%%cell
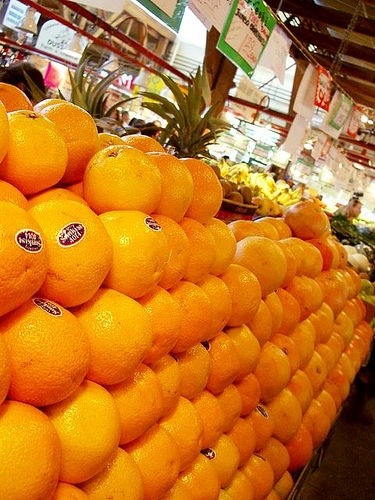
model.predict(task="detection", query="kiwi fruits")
[208,165,252,204]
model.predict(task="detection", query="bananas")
[208,159,327,208]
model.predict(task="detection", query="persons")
[103,109,160,141]
[0,63,47,107]
[298,182,309,200]
[334,198,361,223]
